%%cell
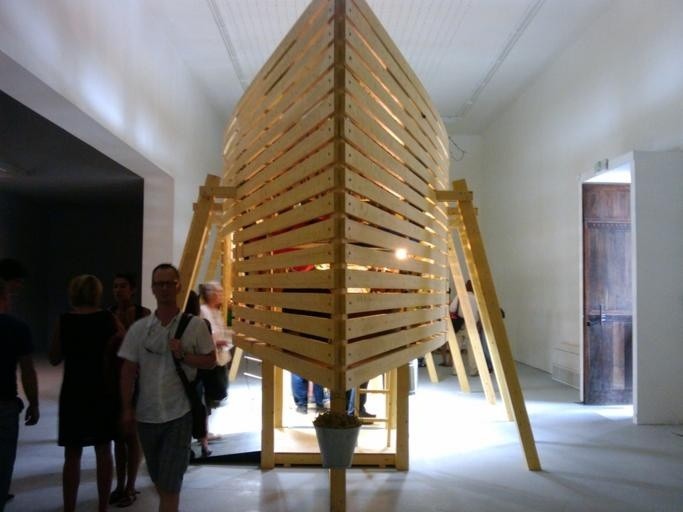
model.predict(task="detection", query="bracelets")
[178,349,185,360]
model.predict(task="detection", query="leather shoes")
[351,412,376,425]
[296,406,327,413]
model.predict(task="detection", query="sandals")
[110,488,137,507]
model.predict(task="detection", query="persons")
[0,282,39,512]
[185,279,236,462]
[289,370,330,415]
[346,378,377,425]
[474,319,492,373]
[450,279,482,377]
[114,262,218,512]
[96,272,150,510]
[43,274,126,512]
[436,286,456,367]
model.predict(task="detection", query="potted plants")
[313,412,363,469]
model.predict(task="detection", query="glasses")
[155,280,177,288]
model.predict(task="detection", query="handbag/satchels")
[451,314,464,333]
[185,378,208,414]
